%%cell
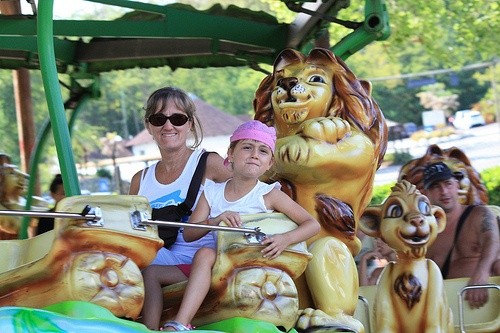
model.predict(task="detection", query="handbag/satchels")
[151,151,217,247]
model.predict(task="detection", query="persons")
[137,121,321,331]
[423,162,500,309]
[31,174,66,237]
[353,230,376,264]
[359,238,398,286]
[127,87,233,279]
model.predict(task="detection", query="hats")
[424,162,451,190]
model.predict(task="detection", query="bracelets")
[207,218,211,225]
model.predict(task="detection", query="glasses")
[146,113,191,126]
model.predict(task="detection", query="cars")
[447,110,485,129]
[384,119,437,140]
